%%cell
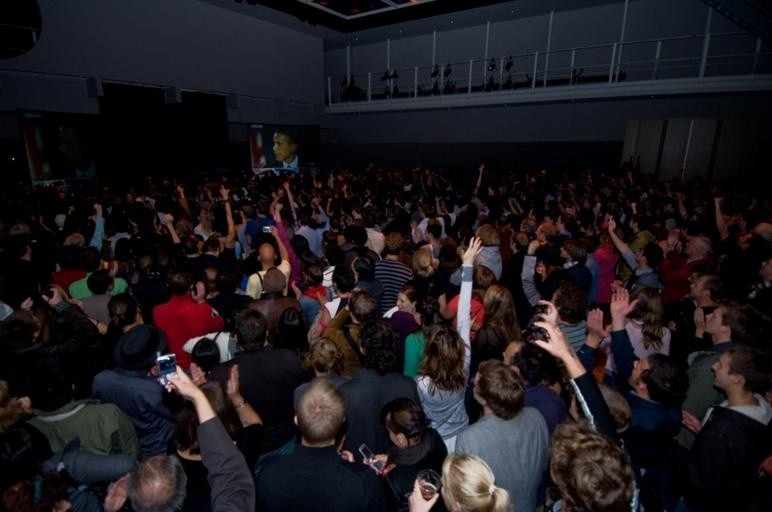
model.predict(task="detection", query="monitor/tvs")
[247,122,321,175]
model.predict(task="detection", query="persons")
[1,167,770,511]
[273,127,309,170]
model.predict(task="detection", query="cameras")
[518,305,546,344]
[263,226,272,233]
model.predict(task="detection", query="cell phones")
[610,215,614,224]
[156,353,178,386]
[358,443,386,474]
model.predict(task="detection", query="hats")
[262,267,286,293]
[119,323,166,370]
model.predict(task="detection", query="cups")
[414,469,441,500]
